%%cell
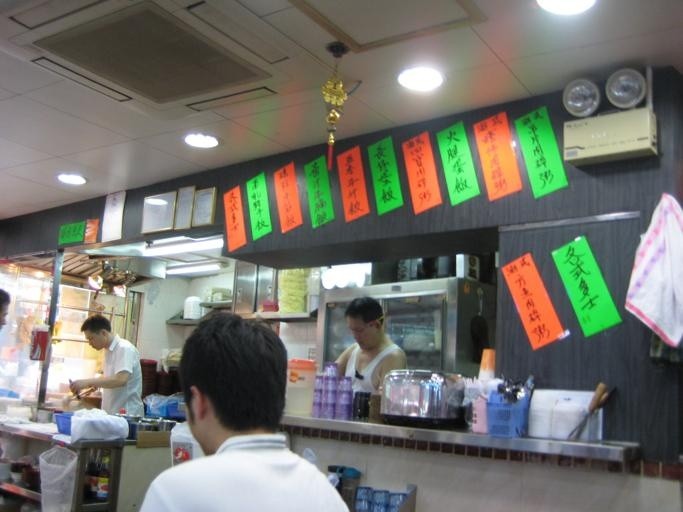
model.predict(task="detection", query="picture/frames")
[173,185,198,233]
[190,186,218,228]
[140,189,178,234]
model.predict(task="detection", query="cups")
[353,391,370,419]
[316,361,352,417]
[354,486,408,512]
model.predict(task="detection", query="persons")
[69,314,144,419]
[329,297,409,395]
[0,289,9,330]
[137,312,350,512]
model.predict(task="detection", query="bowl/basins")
[10,471,23,483]
[146,418,176,429]
[11,461,26,472]
[116,414,141,421]
[131,423,158,439]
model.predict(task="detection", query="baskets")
[486,391,528,439]
[54,412,73,435]
[145,398,187,418]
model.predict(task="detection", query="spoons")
[495,378,528,405]
[63,385,95,408]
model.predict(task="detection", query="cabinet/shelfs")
[0,424,123,510]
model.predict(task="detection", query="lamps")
[89,274,105,292]
[114,283,128,299]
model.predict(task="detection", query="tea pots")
[284,358,318,415]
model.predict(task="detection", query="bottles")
[97,457,109,501]
[471,349,497,434]
[86,447,97,498]
[340,469,360,512]
[326,465,343,492]
